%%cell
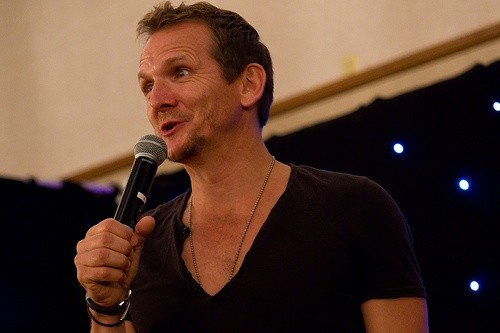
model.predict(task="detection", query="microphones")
[114,135,168,234]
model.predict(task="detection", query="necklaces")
[187,156,276,288]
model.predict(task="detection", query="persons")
[73,3,429,333]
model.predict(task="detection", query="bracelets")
[84,288,132,315]
[86,304,130,327]
